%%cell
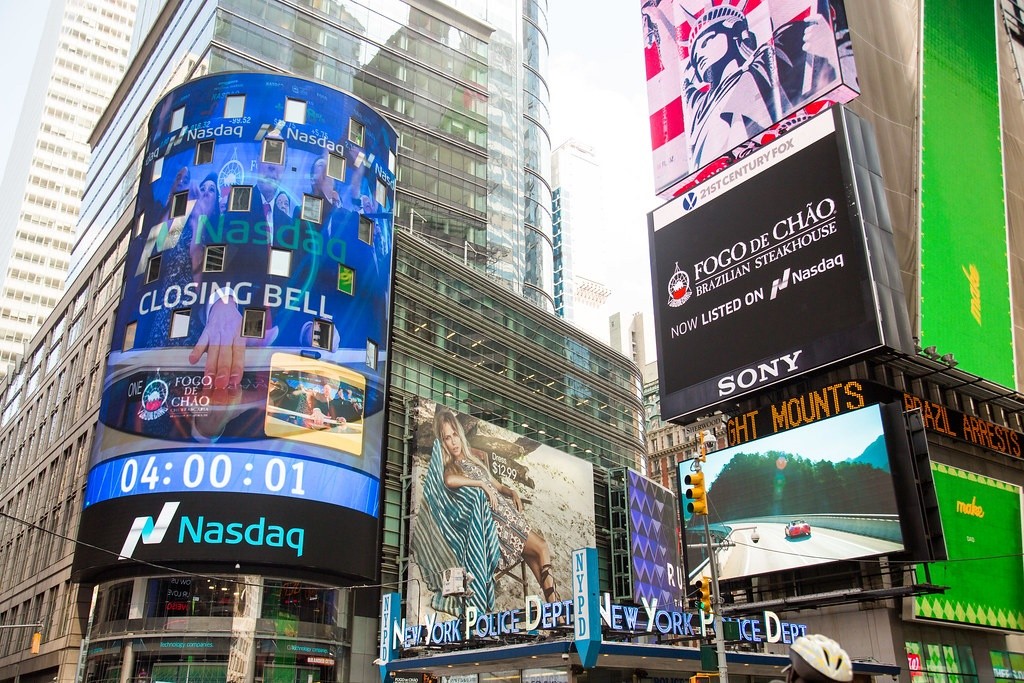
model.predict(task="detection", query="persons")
[432,407,571,637]
[782,633,854,683]
[87,673,97,683]
[132,134,391,431]
[138,667,149,683]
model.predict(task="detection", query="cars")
[785,520,811,538]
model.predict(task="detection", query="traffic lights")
[696,577,710,612]
[31,632,41,654]
[685,472,708,515]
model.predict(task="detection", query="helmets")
[789,634,853,683]
